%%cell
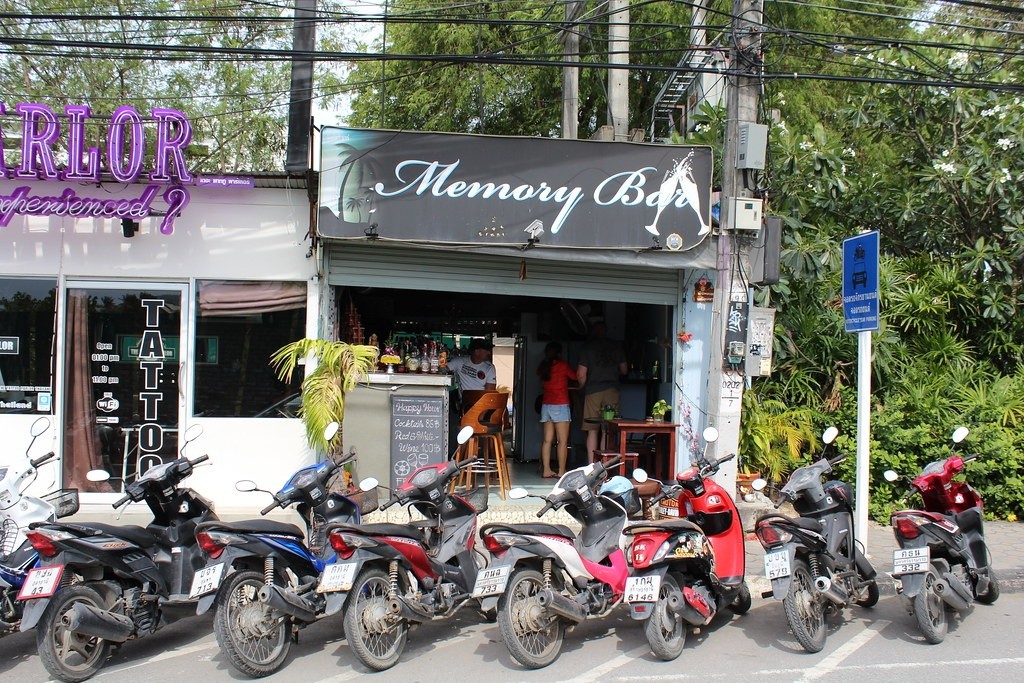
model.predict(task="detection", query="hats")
[470,339,495,350]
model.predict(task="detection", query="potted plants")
[652,399,672,423]
[384,331,398,354]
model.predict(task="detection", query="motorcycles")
[748,427,879,655]
[0,417,80,641]
[9,427,225,683]
[183,423,363,677]
[317,424,487,672]
[471,450,637,669]
[884,426,1001,644]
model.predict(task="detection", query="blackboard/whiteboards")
[390,394,449,496]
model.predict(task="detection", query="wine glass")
[679,170,709,235]
[644,170,678,236]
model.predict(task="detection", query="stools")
[593,450,638,479]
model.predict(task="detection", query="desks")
[601,419,680,485]
[342,372,451,499]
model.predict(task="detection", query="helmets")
[598,475,635,507]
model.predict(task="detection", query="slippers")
[543,473,561,478]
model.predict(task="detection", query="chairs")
[447,391,511,499]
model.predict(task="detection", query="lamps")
[364,224,379,239]
[639,236,662,254]
[520,219,544,251]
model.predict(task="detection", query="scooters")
[615,426,752,662]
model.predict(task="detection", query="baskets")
[41,489,79,518]
[456,487,488,514]
[827,483,853,511]
[609,488,640,514]
[348,486,379,518]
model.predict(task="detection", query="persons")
[537,342,577,479]
[578,320,627,466]
[444,338,498,477]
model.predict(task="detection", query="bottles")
[397,337,446,375]
[645,416,654,422]
[652,361,659,379]
